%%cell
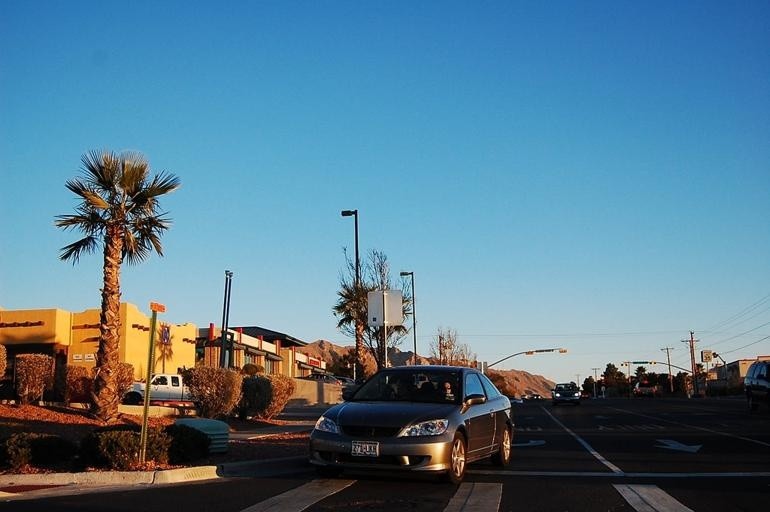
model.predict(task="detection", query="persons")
[438,380,455,400]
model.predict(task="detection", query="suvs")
[632,381,656,398]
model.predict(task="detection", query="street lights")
[399,270,419,366]
[341,209,360,291]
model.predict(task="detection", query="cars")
[305,363,513,482]
[550,381,582,403]
[743,359,770,411]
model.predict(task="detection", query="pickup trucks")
[121,373,212,407]
